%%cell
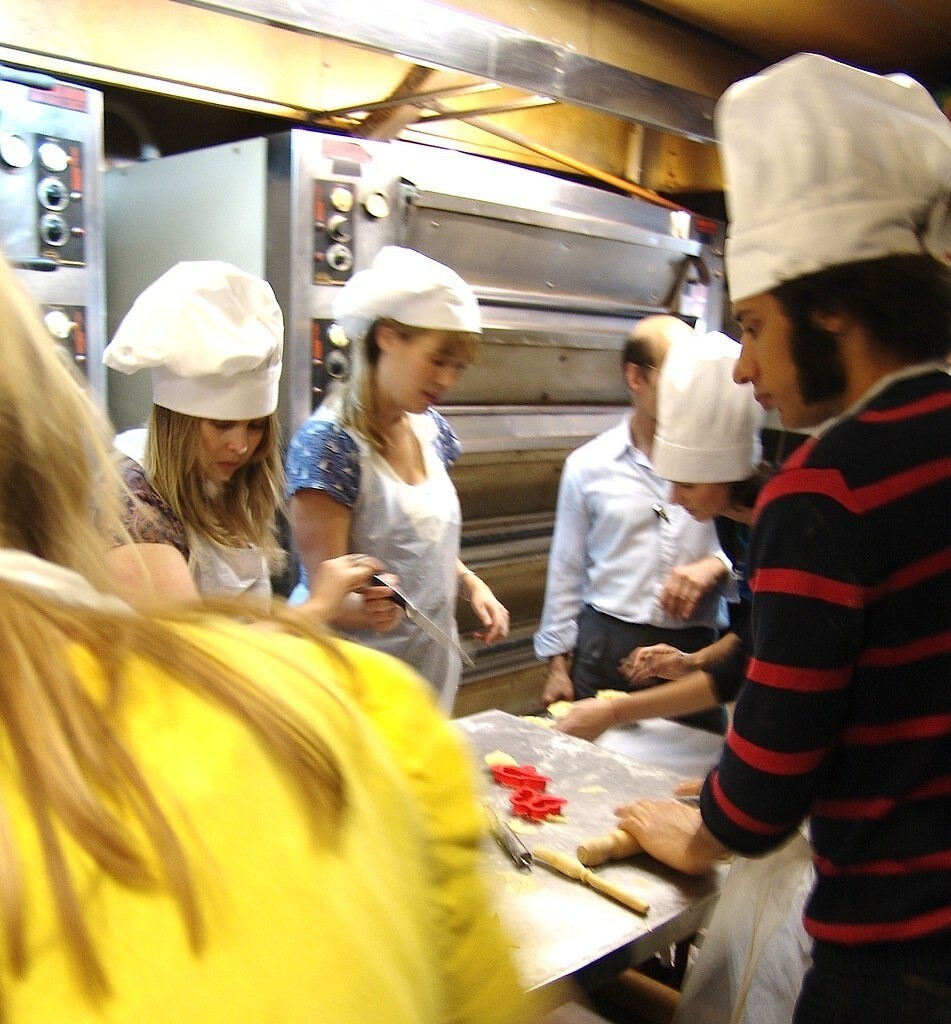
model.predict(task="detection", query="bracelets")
[457,569,475,603]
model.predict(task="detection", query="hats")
[331,246,483,335]
[650,331,762,483]
[104,261,285,420]
[713,52,951,305]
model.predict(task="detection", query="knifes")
[376,574,477,671]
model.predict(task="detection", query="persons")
[0,249,534,1024]
[284,243,510,718]
[611,50,951,1024]
[90,260,384,635]
[552,332,783,741]
[530,314,741,741]
